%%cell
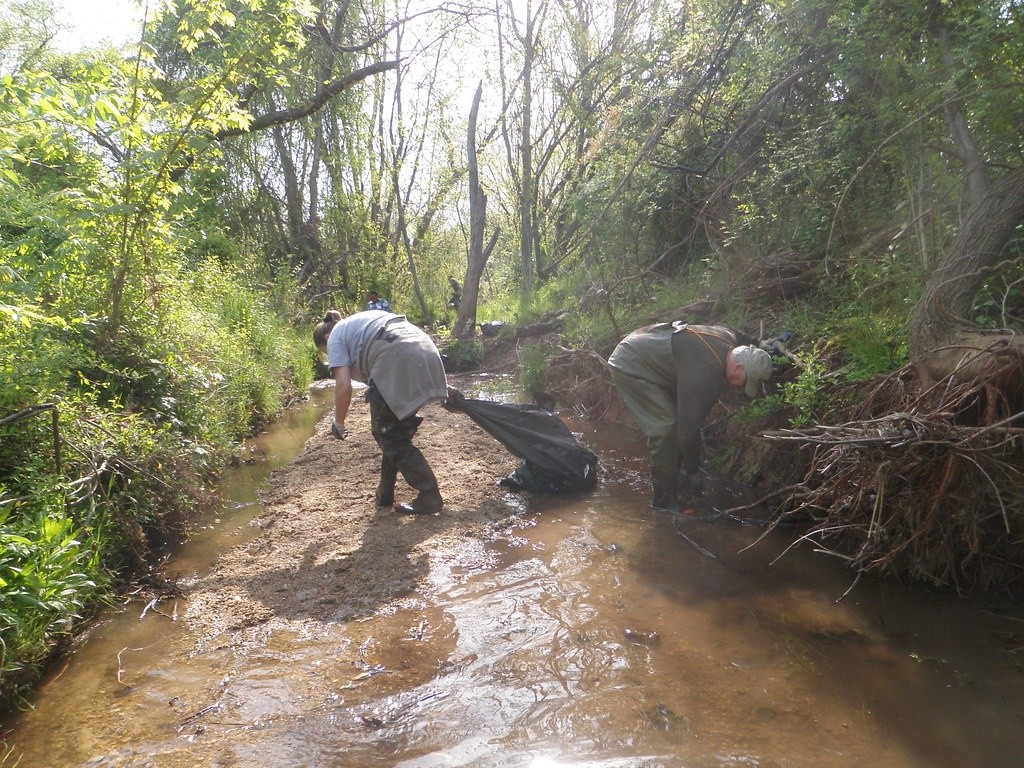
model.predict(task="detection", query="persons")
[368,292,389,313]
[313,310,449,515]
[607,320,779,516]
[448,276,461,305]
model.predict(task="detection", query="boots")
[394,451,443,515]
[651,467,690,512]
[376,452,398,506]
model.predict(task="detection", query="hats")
[744,344,772,399]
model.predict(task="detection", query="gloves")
[441,384,464,414]
[332,419,348,441]
